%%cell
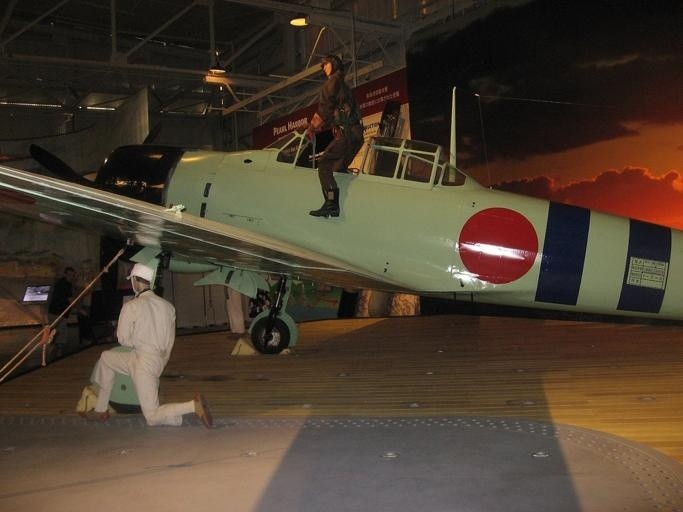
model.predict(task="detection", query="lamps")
[208,51,227,75]
[289,11,309,27]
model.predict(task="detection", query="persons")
[47,267,87,362]
[308,51,365,218]
[83,264,213,426]
[222,285,246,342]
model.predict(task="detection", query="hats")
[125,263,154,283]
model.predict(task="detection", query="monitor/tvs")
[21,283,54,305]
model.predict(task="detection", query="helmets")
[319,55,345,73]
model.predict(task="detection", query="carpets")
[0,404,683,512]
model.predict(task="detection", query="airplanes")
[0,86,683,357]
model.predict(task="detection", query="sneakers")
[85,408,111,422]
[191,391,212,429]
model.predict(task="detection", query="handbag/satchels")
[75,384,115,419]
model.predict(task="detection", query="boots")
[309,188,339,217]
